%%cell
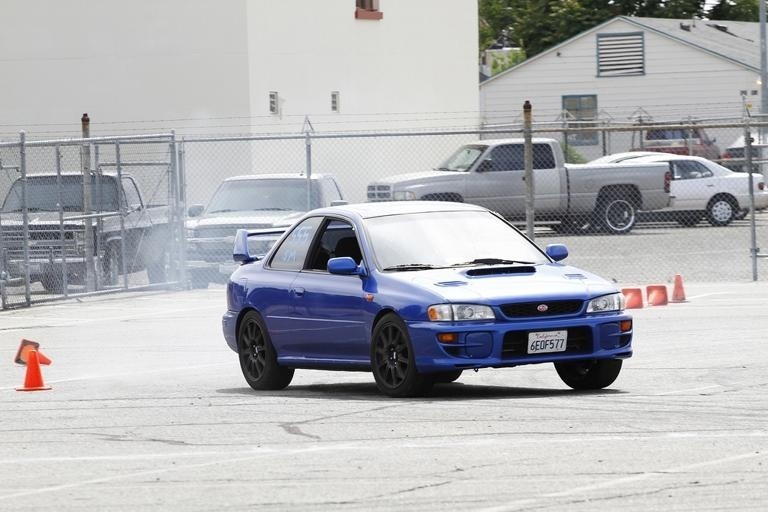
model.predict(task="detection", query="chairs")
[335,237,363,265]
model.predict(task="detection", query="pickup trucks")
[627,123,721,166]
[1,170,191,294]
[363,136,673,235]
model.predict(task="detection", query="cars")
[723,132,765,176]
[552,152,768,233]
[217,197,634,401]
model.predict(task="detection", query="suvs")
[176,169,348,291]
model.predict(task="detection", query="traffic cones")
[13,336,53,370]
[14,349,53,392]
[646,284,668,307]
[667,272,690,304]
[620,286,644,312]
